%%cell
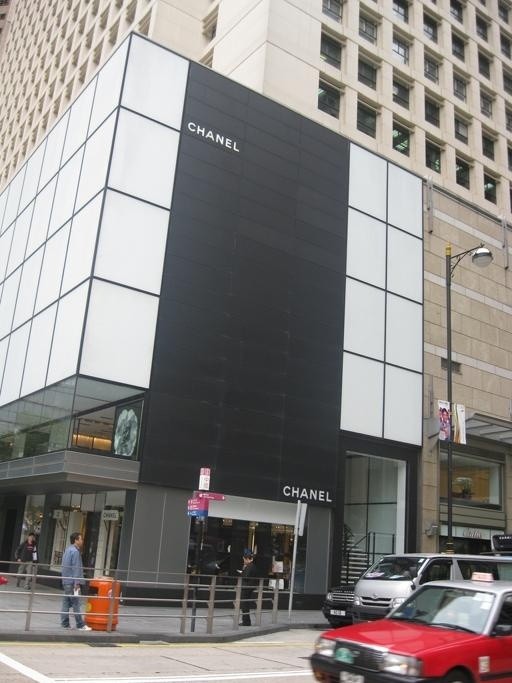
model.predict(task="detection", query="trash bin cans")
[84,576,120,631]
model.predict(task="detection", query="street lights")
[445,242,493,545]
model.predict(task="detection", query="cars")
[311,553,512,682]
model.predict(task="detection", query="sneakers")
[239,621,252,626]
[16,582,32,590]
[78,624,92,631]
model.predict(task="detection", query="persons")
[60,531,93,631]
[439,407,449,440]
[238,552,262,626]
[283,554,292,589]
[15,532,39,589]
